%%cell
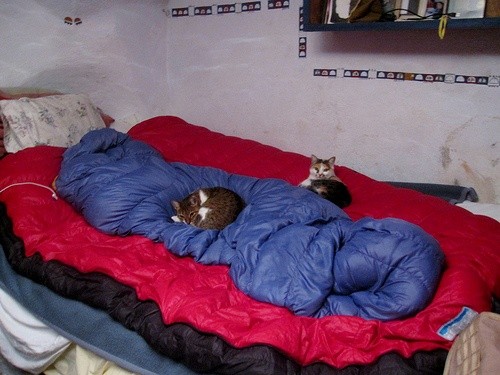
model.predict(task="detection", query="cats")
[170,186,246,230]
[295,154,352,210]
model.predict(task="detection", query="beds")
[0,91,500,375]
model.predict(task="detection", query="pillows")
[0,93,107,154]
